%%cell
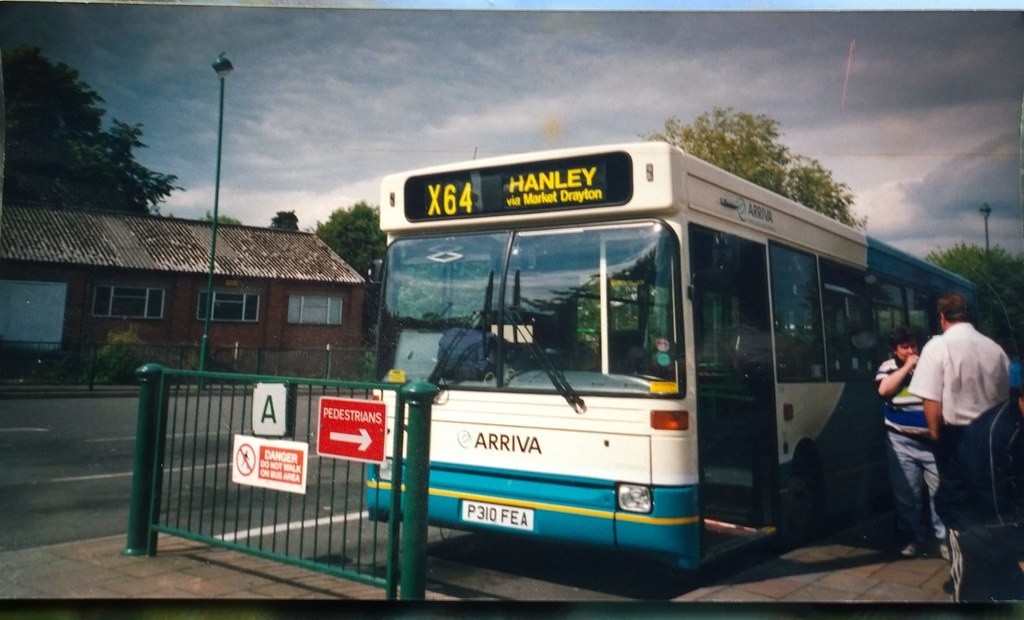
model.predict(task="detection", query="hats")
[1008,362,1024,387]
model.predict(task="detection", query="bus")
[362,139,980,574]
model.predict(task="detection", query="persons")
[933,390,1024,601]
[875,332,949,559]
[906,294,1009,595]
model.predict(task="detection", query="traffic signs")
[315,396,387,463]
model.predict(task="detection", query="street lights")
[194,54,236,390]
[979,201,992,255]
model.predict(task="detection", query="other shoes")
[939,544,951,560]
[902,543,917,557]
